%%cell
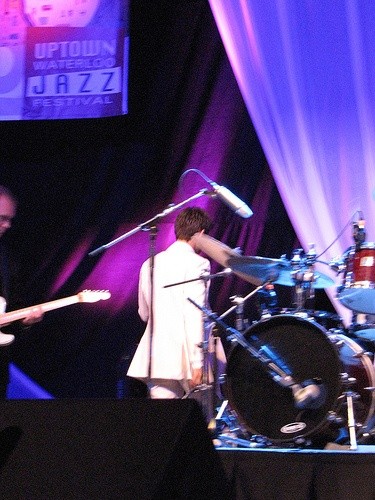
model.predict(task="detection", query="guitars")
[0,289,111,346]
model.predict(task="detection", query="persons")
[0,185,44,400]
[127,207,227,445]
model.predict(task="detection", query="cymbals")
[224,255,335,289]
[191,232,264,287]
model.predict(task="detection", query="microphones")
[194,377,227,393]
[206,268,233,280]
[207,180,254,218]
[293,385,322,408]
[353,211,366,246]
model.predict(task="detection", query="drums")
[221,312,375,450]
[335,242,375,315]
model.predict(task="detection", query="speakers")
[0,396,237,500]
